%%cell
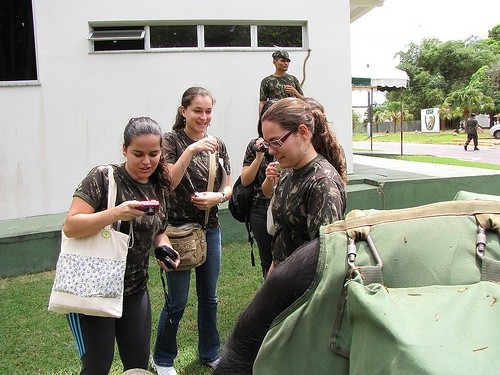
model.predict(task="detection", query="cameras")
[274,162,284,170]
[135,200,160,216]
[260,143,268,152]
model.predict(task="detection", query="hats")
[471,113,476,116]
[272,50,291,63]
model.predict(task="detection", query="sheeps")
[120,200,500,375]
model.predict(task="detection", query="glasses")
[263,129,297,149]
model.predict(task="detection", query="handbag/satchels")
[227,174,255,223]
[164,221,208,271]
[47,165,130,318]
[267,186,278,236]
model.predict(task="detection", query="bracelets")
[219,191,227,204]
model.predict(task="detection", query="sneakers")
[207,357,221,370]
[151,357,178,375]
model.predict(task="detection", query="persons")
[262,99,346,281]
[63,117,181,375]
[150,88,233,375]
[242,100,279,281]
[464,113,484,150]
[258,51,305,125]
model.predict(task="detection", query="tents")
[351,63,409,158]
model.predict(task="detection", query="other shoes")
[464,145,467,150]
[474,148,480,150]
